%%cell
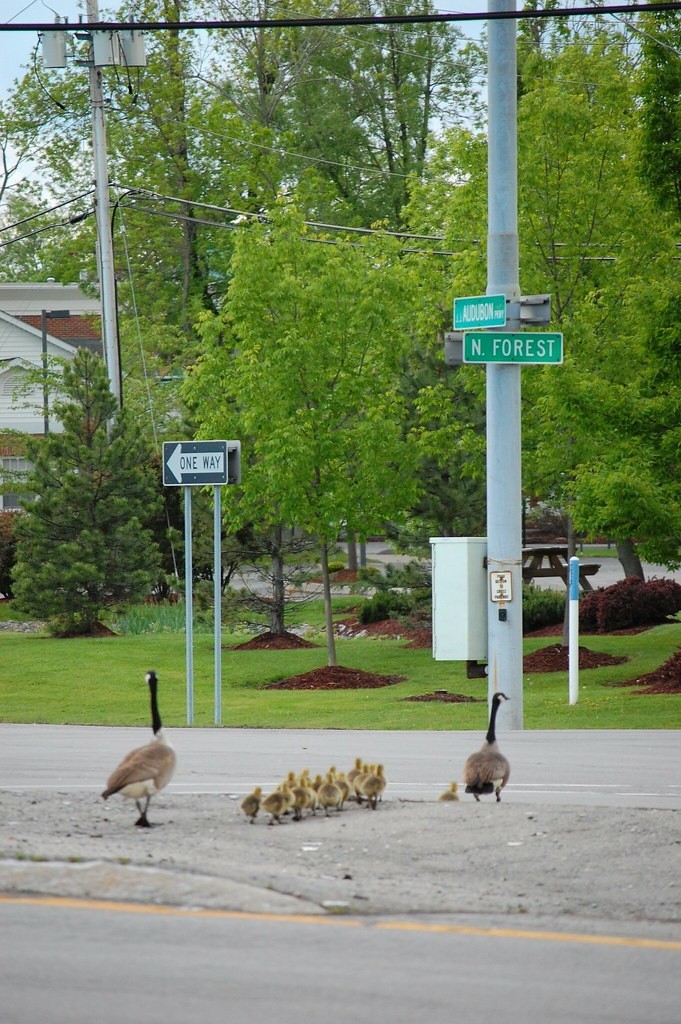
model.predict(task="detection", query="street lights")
[40,308,71,436]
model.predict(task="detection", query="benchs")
[523,511,601,591]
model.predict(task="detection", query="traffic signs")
[162,441,228,486]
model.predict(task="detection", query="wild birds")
[240,755,388,827]
[435,691,512,803]
[99,670,178,829]
